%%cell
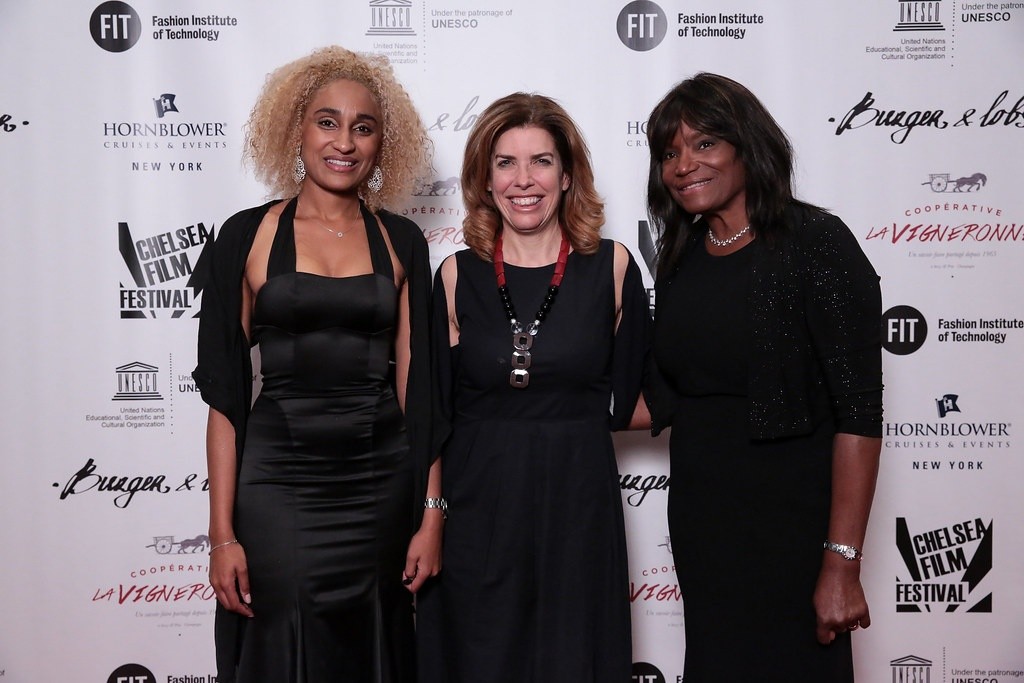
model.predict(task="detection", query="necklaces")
[296,193,360,240]
[707,224,750,247]
[492,224,570,389]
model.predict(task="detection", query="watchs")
[423,497,449,520]
[823,537,862,562]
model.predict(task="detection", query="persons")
[629,73,884,683]
[185,46,450,683]
[429,94,652,683]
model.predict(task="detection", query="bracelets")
[207,537,238,556]
